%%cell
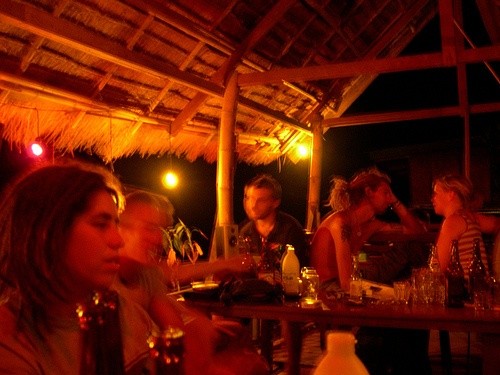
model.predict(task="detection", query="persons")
[0,162,273,375]
[234,169,500,375]
[112,191,272,375]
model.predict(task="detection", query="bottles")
[256,237,273,293]
[346,255,363,305]
[444,239,465,308]
[281,247,300,300]
[428,245,441,306]
[147,327,185,375]
[468,238,487,304]
[315,332,369,375]
[78,288,125,375]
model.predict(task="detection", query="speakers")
[215,225,240,260]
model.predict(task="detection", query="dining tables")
[178,289,500,375]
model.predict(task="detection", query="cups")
[474,277,496,314]
[392,281,411,304]
[237,235,253,273]
[411,267,429,304]
[300,266,320,310]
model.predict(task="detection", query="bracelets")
[389,199,401,211]
